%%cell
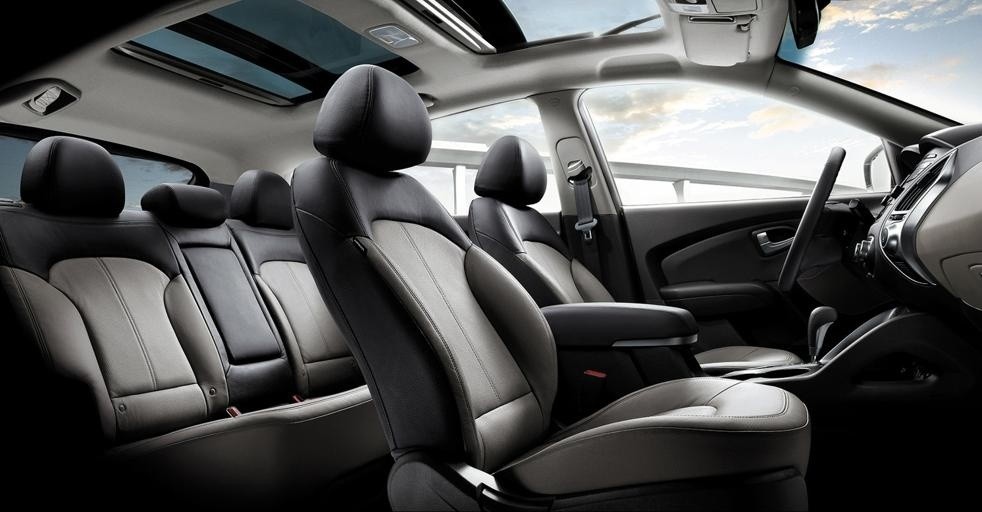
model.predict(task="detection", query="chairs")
[291,64,812,512]
[467,134,803,395]
